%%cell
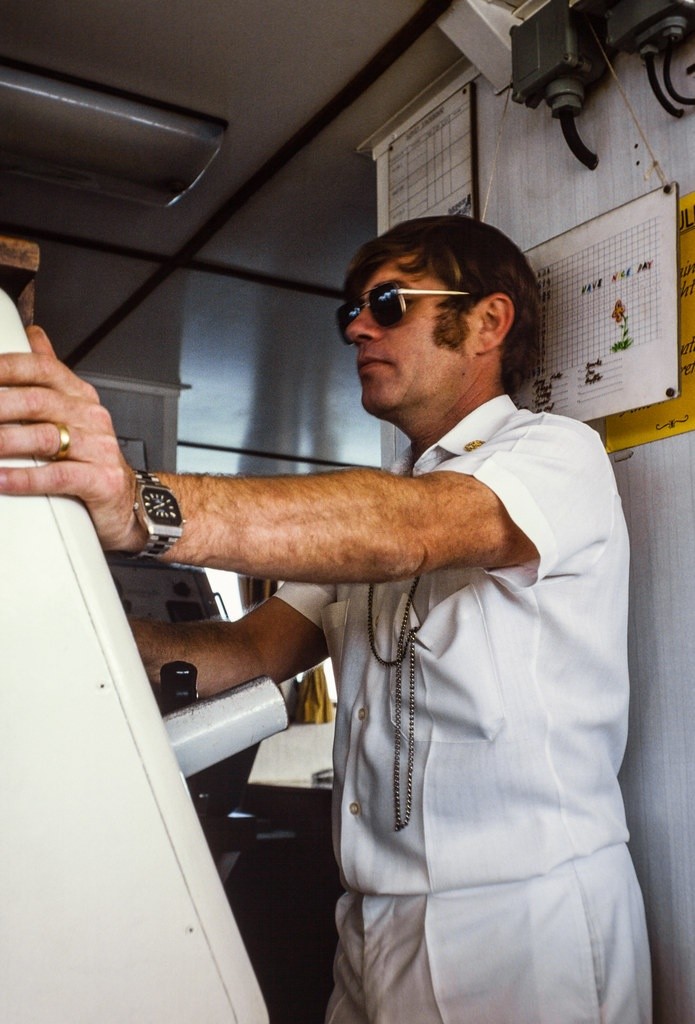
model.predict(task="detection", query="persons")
[1,214,654,1024]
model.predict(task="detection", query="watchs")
[118,468,186,567]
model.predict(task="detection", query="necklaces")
[365,468,420,831]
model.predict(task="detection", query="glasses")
[335,282,470,346]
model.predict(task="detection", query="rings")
[51,421,71,460]
